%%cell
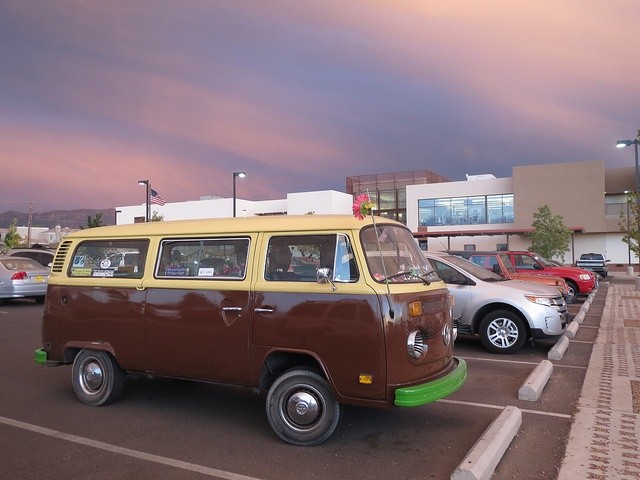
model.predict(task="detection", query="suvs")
[4,248,56,266]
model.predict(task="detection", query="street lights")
[616,140,639,226]
[233,173,246,216]
[138,180,148,221]
[115,210,122,225]
[624,190,631,266]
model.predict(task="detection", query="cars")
[341,251,567,355]
[577,253,610,279]
[458,253,573,304]
[509,252,597,302]
[0,257,50,304]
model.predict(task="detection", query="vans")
[34,215,468,446]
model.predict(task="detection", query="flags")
[151,190,166,206]
